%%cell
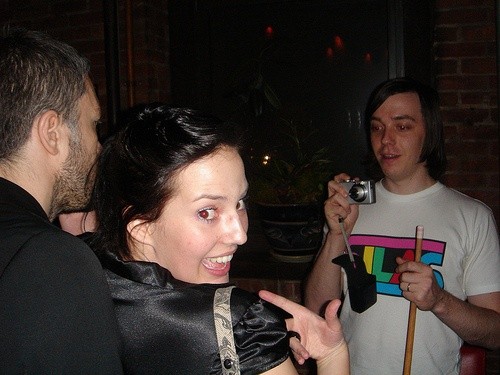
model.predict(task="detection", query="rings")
[287,330,301,341]
[407,283,410,292]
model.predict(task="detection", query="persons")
[301,74,500,375]
[0,27,126,375]
[58,105,352,375]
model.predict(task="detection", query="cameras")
[339,179,377,204]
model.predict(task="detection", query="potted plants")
[238,85,333,263]
